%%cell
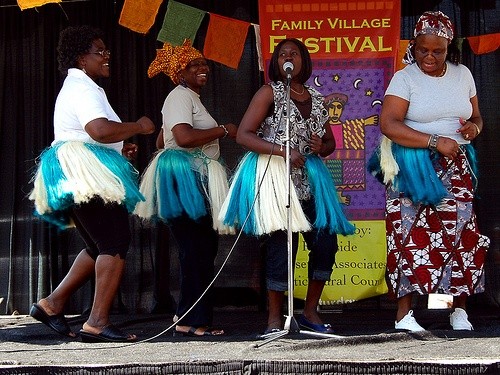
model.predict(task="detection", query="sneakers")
[449,308,474,331]
[395,310,425,331]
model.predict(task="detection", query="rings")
[465,133,469,139]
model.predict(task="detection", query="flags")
[119,0,163,35]
[467,32,500,55]
[398,40,412,71]
[157,0,206,48]
[253,23,265,70]
[202,13,250,68]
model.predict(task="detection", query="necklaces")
[424,62,445,77]
[283,83,305,95]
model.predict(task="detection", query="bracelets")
[473,122,480,137]
[429,133,438,149]
[218,124,228,139]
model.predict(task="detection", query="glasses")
[86,49,111,57]
[415,42,448,58]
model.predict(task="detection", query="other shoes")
[299,314,334,334]
[264,326,282,334]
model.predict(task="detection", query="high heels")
[30,303,78,338]
[80,324,139,343]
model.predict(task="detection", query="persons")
[21,25,156,342]
[132,37,238,337]
[235,37,356,339]
[364,11,487,338]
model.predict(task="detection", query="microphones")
[282,61,295,79]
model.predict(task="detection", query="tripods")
[254,80,344,348]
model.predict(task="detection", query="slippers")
[174,325,224,336]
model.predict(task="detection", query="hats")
[147,38,204,85]
